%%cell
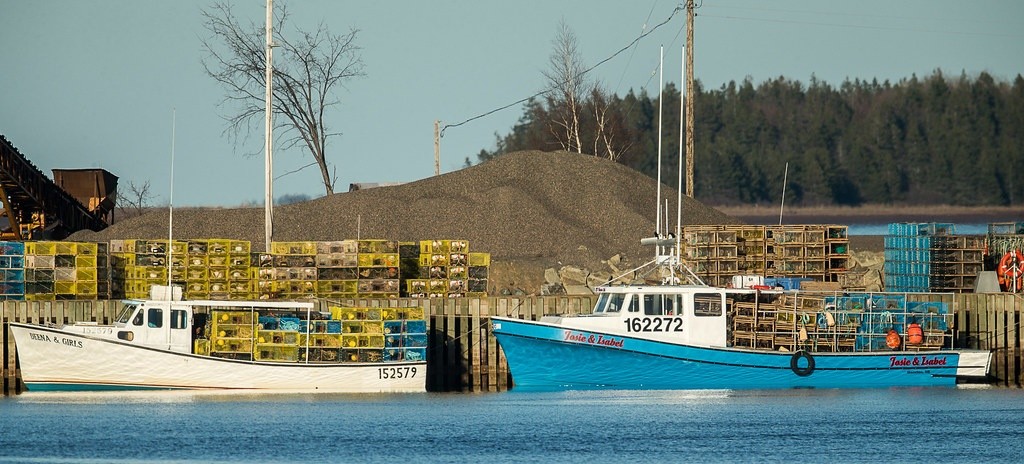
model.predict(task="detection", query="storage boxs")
[677,223,1024,352]
[0,238,489,301]
[194,306,428,362]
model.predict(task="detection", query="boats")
[10,112,428,393]
[491,46,991,391]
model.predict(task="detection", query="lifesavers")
[1002,252,1024,277]
[750,285,775,289]
[791,351,815,376]
